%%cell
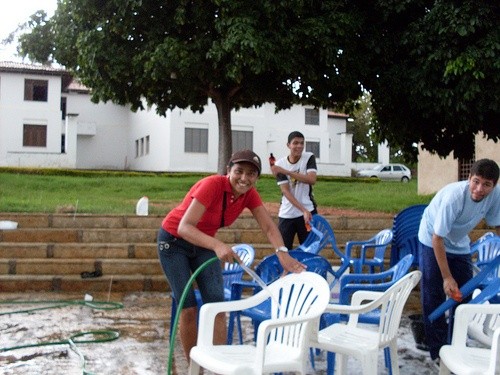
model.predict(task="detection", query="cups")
[84,293,93,302]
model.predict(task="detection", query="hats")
[232,150,261,170]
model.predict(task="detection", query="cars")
[355,163,412,185]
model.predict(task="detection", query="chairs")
[170,204,500,375]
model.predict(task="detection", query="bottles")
[136,196,148,215]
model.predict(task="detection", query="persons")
[157,149,308,366]
[269,152,276,167]
[416,158,500,366]
[271,130,317,251]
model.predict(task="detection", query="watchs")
[276,246,288,253]
